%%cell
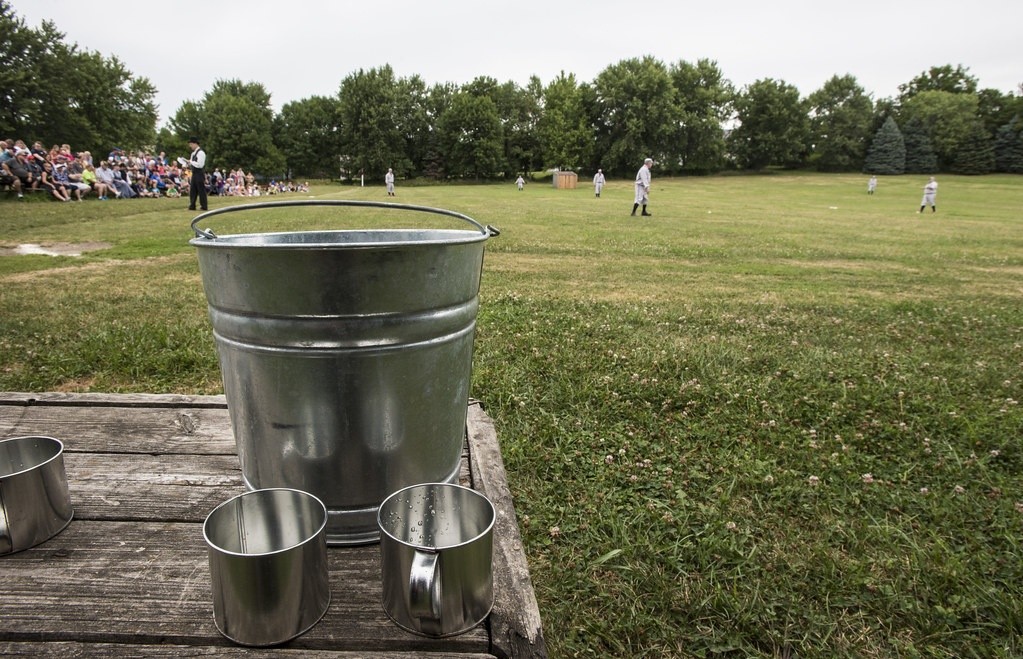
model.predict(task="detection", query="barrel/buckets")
[188,199,500,547]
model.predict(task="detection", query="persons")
[514,174,525,191]
[915,176,938,215]
[593,168,606,197]
[630,158,653,216]
[184,137,207,211]
[0,137,311,202]
[867,175,877,195]
[385,168,395,196]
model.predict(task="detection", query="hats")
[112,147,120,151]
[187,136,200,144]
[54,163,66,169]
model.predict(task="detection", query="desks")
[0,389,545,659]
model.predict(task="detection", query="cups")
[203,488,331,646]
[377,483,496,639]
[0,436,74,556]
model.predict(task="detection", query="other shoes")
[18,192,24,198]
[63,196,71,202]
[116,192,122,199]
[98,195,108,200]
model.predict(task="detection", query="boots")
[388,192,396,196]
[641,204,651,216]
[932,206,936,214]
[631,202,639,216]
[916,205,926,213]
[596,193,600,197]
